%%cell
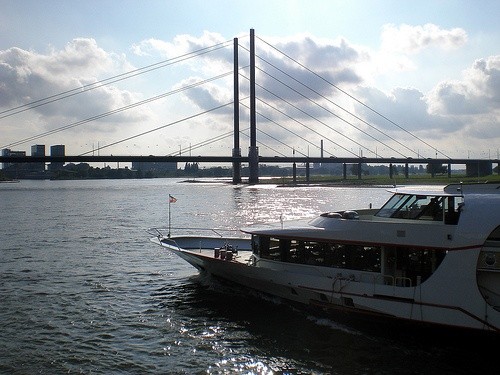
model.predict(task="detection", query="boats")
[145,179,500,357]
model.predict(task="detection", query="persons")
[423,198,442,221]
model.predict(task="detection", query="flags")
[169,196,178,203]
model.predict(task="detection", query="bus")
[7,151,26,157]
